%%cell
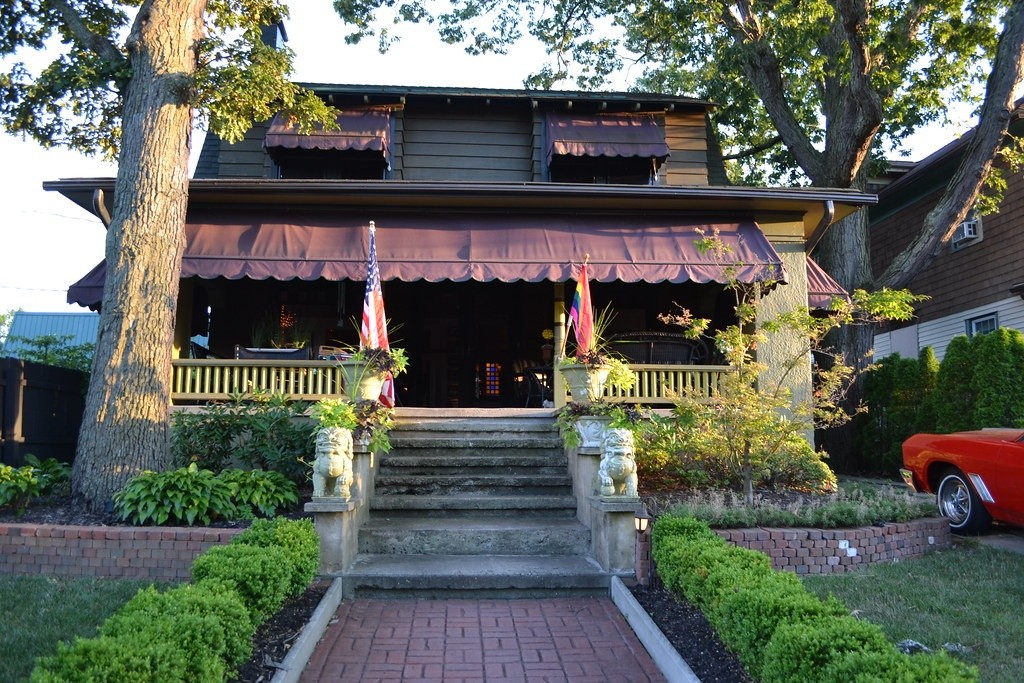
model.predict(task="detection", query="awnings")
[261,104,390,174]
[806,252,852,311]
[179,204,790,284]
[543,107,672,173]
[65,258,107,316]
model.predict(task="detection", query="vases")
[334,360,387,401]
[542,343,552,366]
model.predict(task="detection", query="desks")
[235,344,311,376]
[522,367,555,408]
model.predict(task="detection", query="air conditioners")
[952,221,978,245]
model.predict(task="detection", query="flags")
[570,264,598,362]
[360,229,396,409]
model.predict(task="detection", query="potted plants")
[557,350,637,403]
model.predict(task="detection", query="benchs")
[600,331,710,377]
[190,341,233,377]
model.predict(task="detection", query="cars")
[898,425,1024,540]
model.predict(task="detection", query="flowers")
[352,347,410,379]
[542,329,555,346]
